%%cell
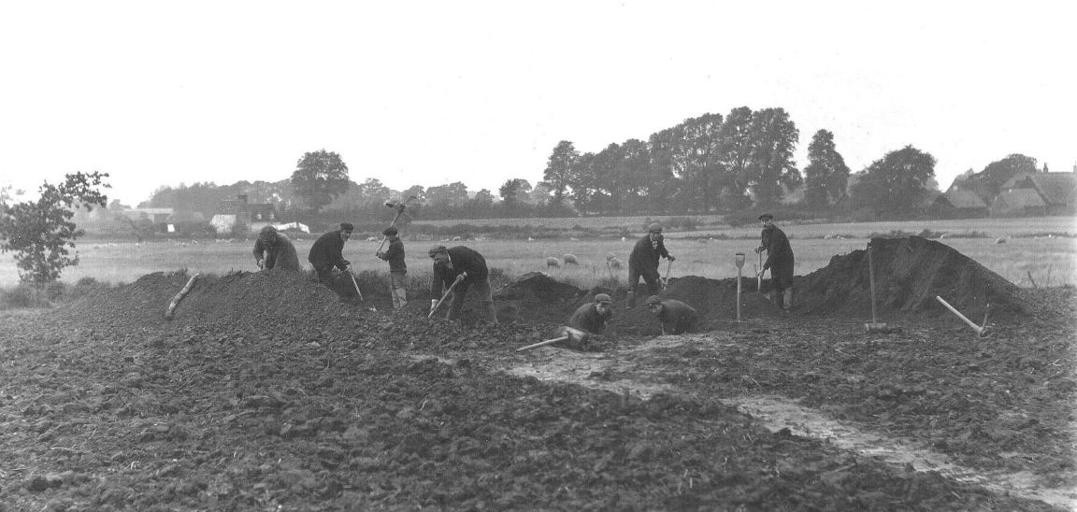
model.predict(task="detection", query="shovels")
[750,250,771,301]
[346,264,377,313]
[730,253,748,324]
[865,242,888,332]
[516,323,585,352]
[417,277,461,333]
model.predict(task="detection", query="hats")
[647,295,660,304]
[430,247,445,256]
[340,223,353,230]
[595,294,612,304]
[648,223,662,233]
[758,214,773,220]
[382,228,397,235]
[260,225,276,245]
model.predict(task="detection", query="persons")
[647,295,698,335]
[570,293,615,335]
[253,227,300,273]
[377,227,407,308]
[309,222,353,285]
[627,223,676,307]
[753,214,795,313]
[428,245,498,334]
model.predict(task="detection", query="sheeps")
[547,257,560,269]
[607,255,616,264]
[609,257,620,269]
[564,254,578,265]
